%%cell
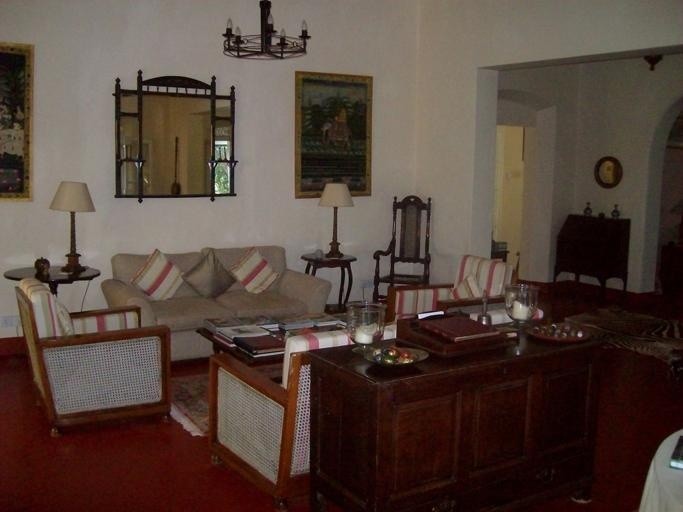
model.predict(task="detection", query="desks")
[491,251,509,263]
[310,320,604,512]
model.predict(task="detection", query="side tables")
[301,253,357,314]
[3,266,100,298]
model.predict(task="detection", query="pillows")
[53,295,73,335]
[131,248,278,302]
[448,275,481,298]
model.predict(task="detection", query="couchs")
[101,247,330,362]
[208,305,542,512]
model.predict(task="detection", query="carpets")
[171,372,207,437]
[566,306,683,377]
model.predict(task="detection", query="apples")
[35,256,50,273]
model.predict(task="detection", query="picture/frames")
[295,70,373,199]
[0,42,34,203]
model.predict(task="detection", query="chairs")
[16,278,171,437]
[373,196,431,301]
[385,256,514,323]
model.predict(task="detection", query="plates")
[364,366,426,380]
[525,327,591,341]
[364,347,429,365]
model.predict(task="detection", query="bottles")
[610,204,619,218]
[620,205,625,219]
[582,201,592,217]
[477,289,494,328]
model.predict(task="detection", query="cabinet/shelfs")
[553,214,631,300]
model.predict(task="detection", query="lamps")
[319,184,354,258]
[223,0,312,59]
[49,181,95,273]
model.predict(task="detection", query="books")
[669,435,682,468]
[204,311,353,357]
[415,314,501,342]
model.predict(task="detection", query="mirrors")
[594,156,622,188]
[113,70,238,203]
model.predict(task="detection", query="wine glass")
[344,301,388,356]
[502,283,541,333]
